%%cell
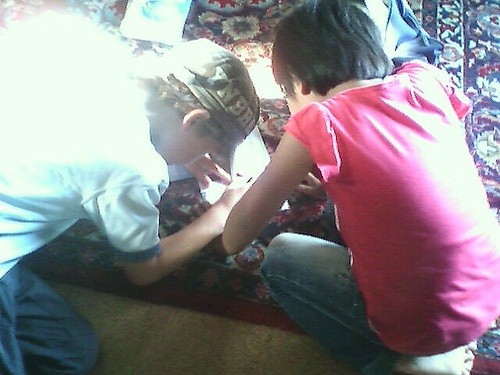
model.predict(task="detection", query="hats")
[164,36,260,151]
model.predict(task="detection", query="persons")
[0,26,274,375]
[214,0,500,374]
